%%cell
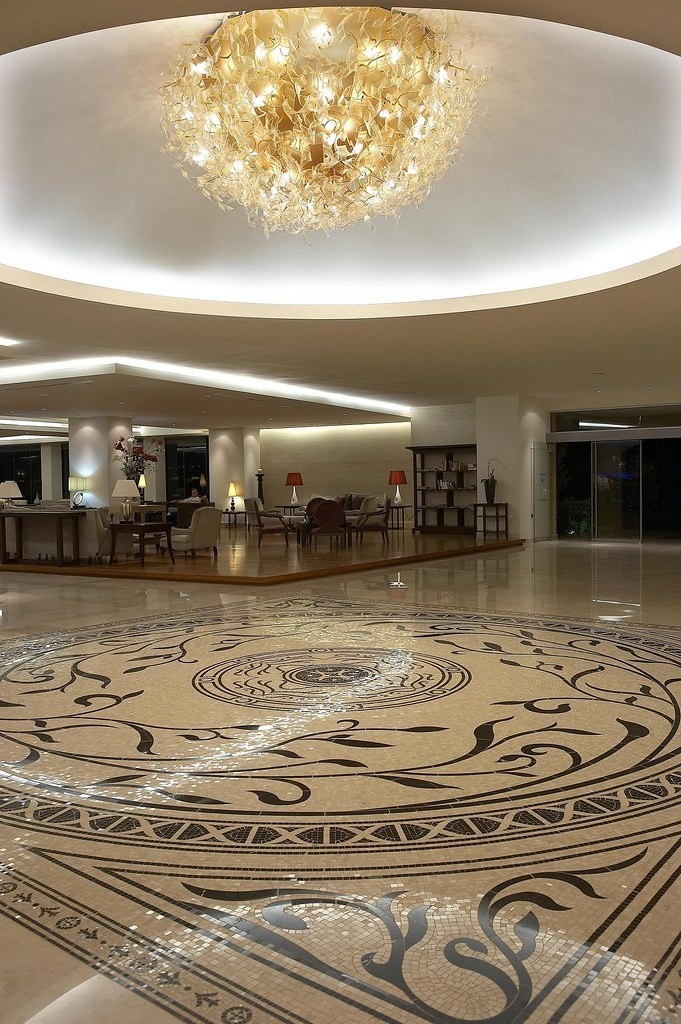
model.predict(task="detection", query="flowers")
[112,433,165,480]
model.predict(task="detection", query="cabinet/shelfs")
[405,444,476,535]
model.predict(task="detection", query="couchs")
[146,501,215,529]
[310,493,388,530]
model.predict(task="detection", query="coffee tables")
[295,521,352,547]
[133,504,167,523]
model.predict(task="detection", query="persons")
[176,489,201,503]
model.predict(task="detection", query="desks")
[0,510,86,567]
[473,503,508,540]
[109,523,175,567]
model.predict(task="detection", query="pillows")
[334,493,367,510]
[200,495,208,502]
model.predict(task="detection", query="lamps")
[200,474,206,488]
[68,476,88,510]
[138,475,147,505]
[388,470,407,506]
[227,482,237,510]
[111,480,140,524]
[156,7,492,245]
[0,483,23,507]
[285,472,303,505]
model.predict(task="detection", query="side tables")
[221,510,248,527]
[386,506,412,530]
[275,505,306,516]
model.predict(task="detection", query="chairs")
[95,507,141,560]
[244,496,392,552]
[158,507,221,558]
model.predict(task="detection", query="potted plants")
[481,458,500,503]
